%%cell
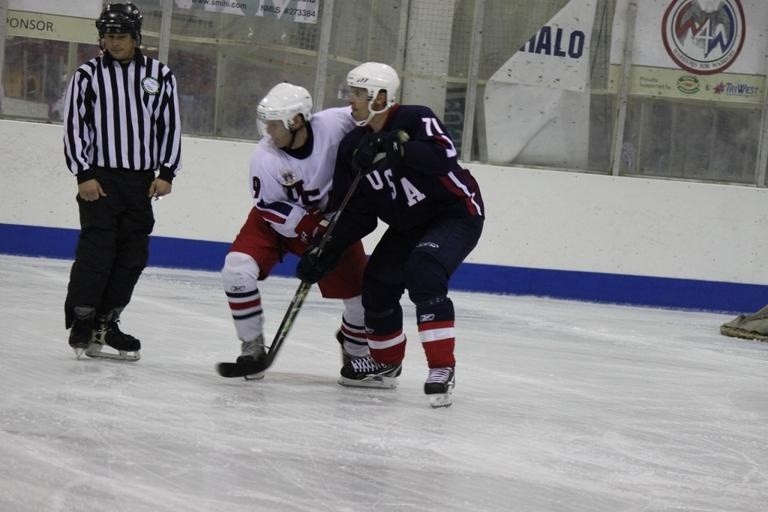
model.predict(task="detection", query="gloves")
[295,244,340,283]
[350,130,402,175]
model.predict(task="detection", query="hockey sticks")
[217,166,366,376]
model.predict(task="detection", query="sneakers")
[236,333,266,363]
[423,366,455,395]
[340,354,403,381]
[68,304,97,349]
[337,329,367,363]
[91,310,142,352]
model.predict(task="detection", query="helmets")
[95,1,143,41]
[345,62,400,115]
[255,82,313,139]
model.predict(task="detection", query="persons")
[220,80,370,367]
[293,60,485,391]
[61,0,182,353]
